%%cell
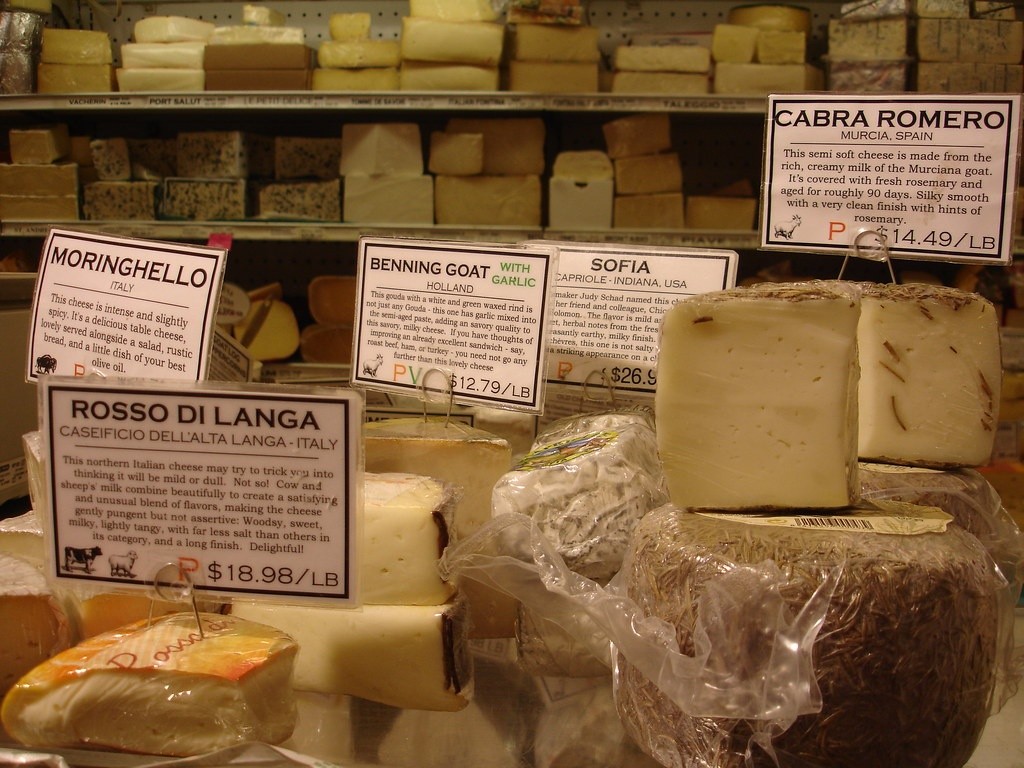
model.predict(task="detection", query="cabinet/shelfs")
[0,0,1024,261]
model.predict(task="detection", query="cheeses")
[0,0,1024,768]
